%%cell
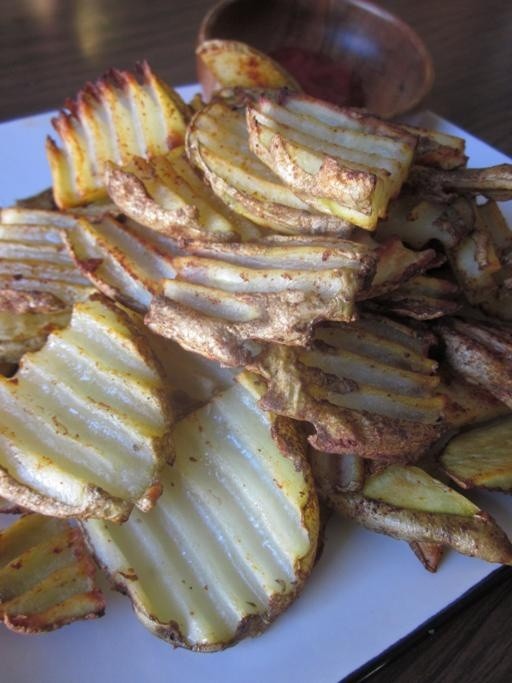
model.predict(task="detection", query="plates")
[1,84,511,681]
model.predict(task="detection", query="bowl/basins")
[197,0,435,124]
